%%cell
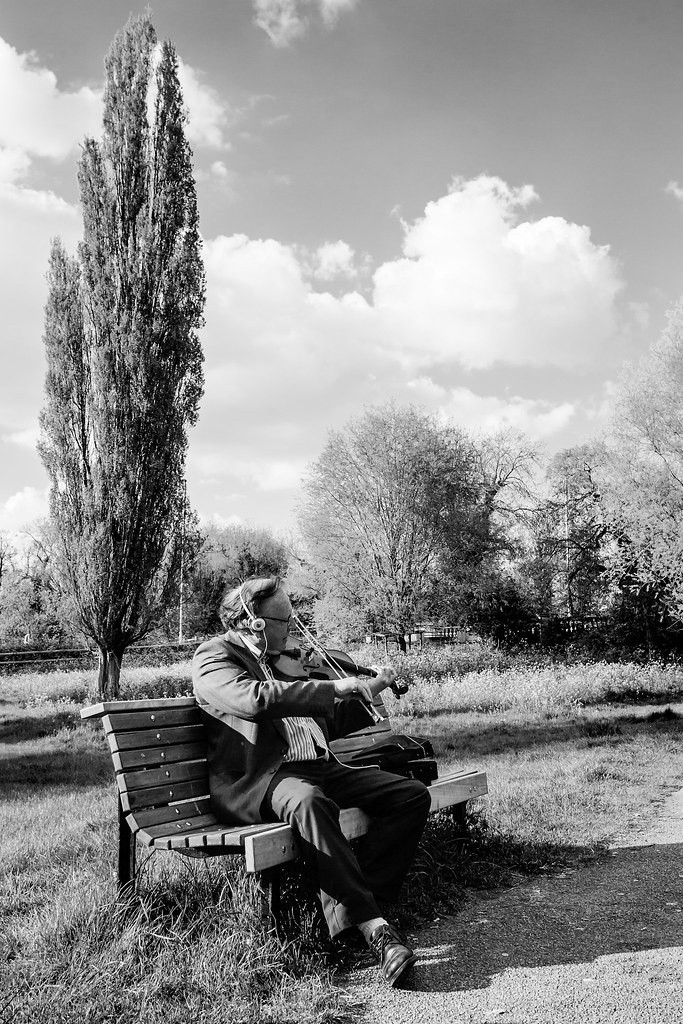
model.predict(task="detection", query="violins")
[270,634,408,700]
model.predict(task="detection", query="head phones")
[239,585,265,631]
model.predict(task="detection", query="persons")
[190,579,431,990]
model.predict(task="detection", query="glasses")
[258,614,292,626]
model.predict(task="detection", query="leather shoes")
[368,924,417,987]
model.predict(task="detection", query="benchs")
[78,693,492,933]
